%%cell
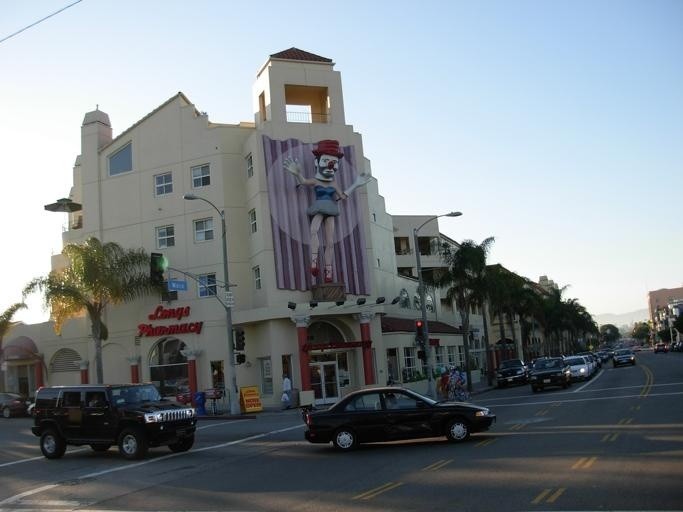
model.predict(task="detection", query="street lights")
[183,193,241,414]
[413,212,463,402]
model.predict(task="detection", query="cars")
[494,359,531,388]
[674,341,683,351]
[563,348,615,382]
[655,343,667,353]
[529,357,571,392]
[304,386,497,450]
[0,392,32,418]
[612,350,636,366]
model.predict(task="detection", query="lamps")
[286,296,400,312]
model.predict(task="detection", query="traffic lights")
[235,328,245,351]
[415,319,424,342]
[150,253,164,284]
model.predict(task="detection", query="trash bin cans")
[194,392,206,416]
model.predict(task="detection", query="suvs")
[31,383,198,460]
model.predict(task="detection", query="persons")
[278,373,293,410]
[281,138,373,286]
[424,363,470,402]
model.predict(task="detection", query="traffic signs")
[168,280,187,290]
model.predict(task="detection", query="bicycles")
[446,375,472,402]
